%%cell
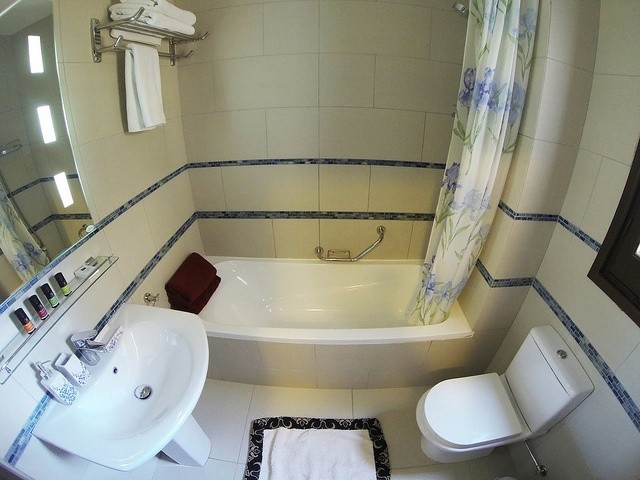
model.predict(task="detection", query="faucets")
[70,330,105,366]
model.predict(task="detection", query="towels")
[108,4,195,36]
[164,251,221,316]
[120,0,197,25]
[124,42,167,133]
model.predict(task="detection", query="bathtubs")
[197,256,473,345]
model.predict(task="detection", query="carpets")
[244,417,391,480]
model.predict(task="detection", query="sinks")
[32,303,209,472]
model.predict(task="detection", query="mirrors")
[0,0,96,304]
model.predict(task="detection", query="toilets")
[415,325,595,464]
